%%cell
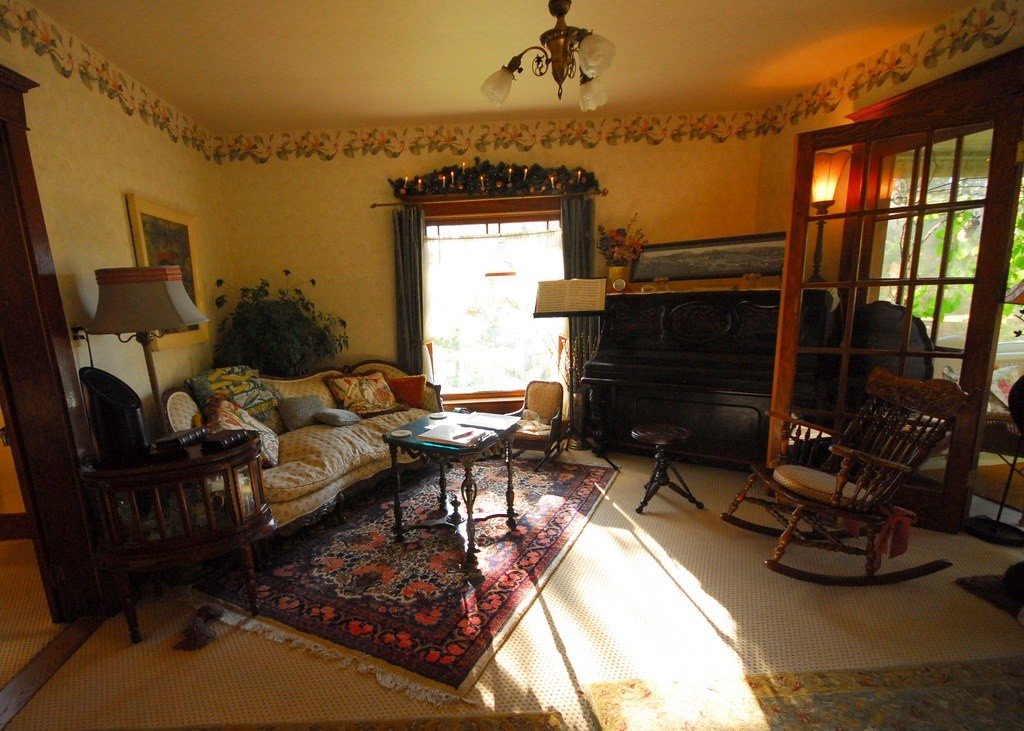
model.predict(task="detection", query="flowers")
[593,210,648,266]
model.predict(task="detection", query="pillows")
[312,408,361,427]
[277,394,326,431]
[389,374,427,408]
[185,365,278,416]
[322,369,410,418]
[205,393,279,467]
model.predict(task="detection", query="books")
[153,427,205,450]
[534,279,606,313]
[416,424,486,446]
[202,430,248,450]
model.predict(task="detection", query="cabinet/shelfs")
[80,429,278,645]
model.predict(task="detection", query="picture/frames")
[124,192,207,352]
[630,231,786,283]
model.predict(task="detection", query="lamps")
[480,0,616,115]
[90,263,210,465]
[807,149,852,281]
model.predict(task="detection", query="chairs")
[503,380,563,459]
[720,365,981,587]
[980,358,1024,456]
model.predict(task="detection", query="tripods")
[532,278,620,473]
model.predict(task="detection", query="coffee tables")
[382,410,526,563]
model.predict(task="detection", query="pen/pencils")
[453,431,474,440]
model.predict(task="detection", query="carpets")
[954,573,1024,626]
[179,455,622,707]
[582,654,1024,731]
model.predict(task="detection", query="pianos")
[581,287,842,475]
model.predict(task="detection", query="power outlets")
[66,321,81,349]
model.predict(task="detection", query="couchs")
[160,358,444,569]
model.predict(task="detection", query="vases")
[607,266,630,293]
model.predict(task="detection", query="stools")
[631,423,704,513]
